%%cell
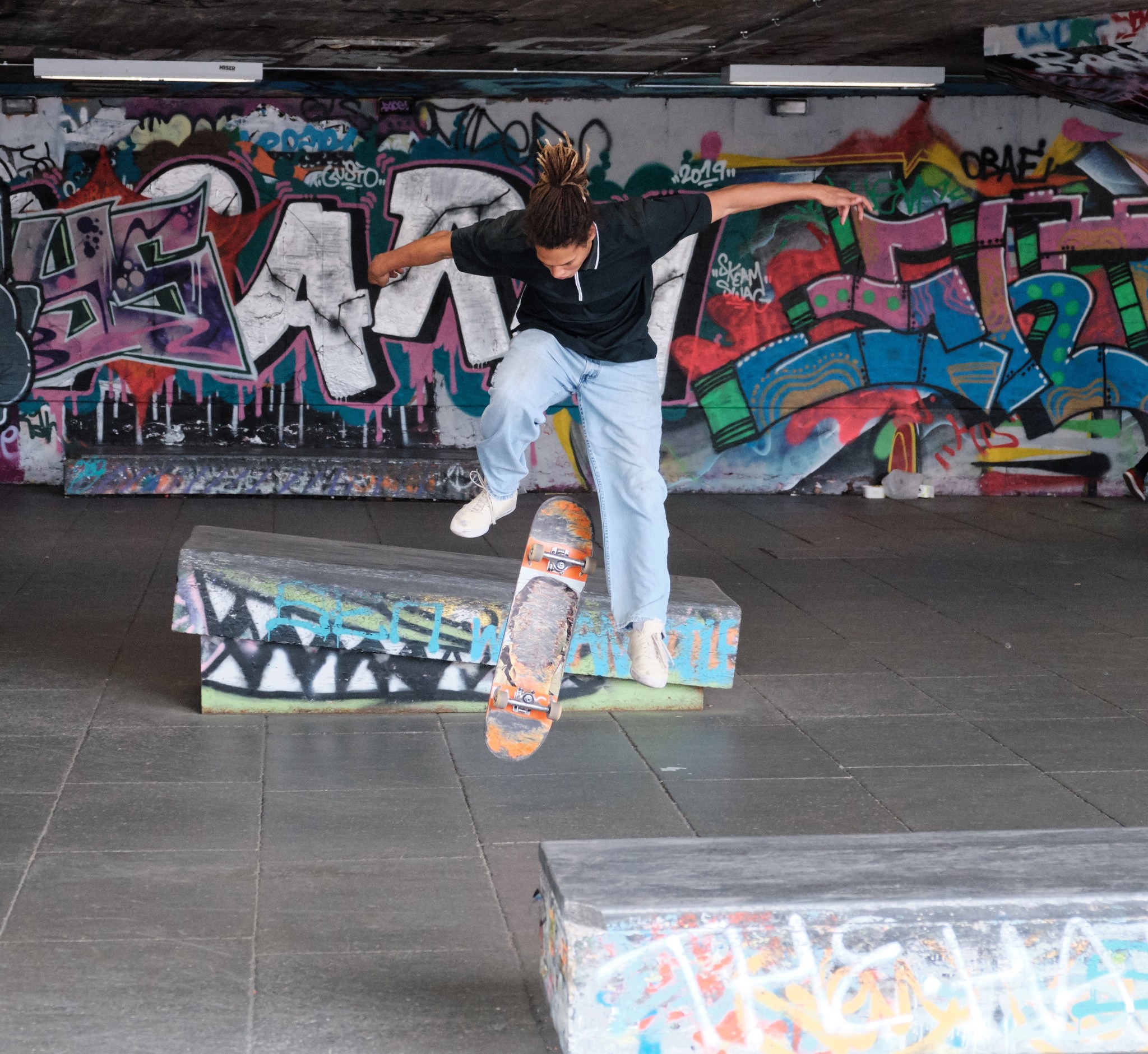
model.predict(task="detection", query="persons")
[364,141,876,690]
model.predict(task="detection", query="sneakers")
[450,469,518,537]
[628,617,676,688]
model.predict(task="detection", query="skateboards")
[483,491,597,763]
